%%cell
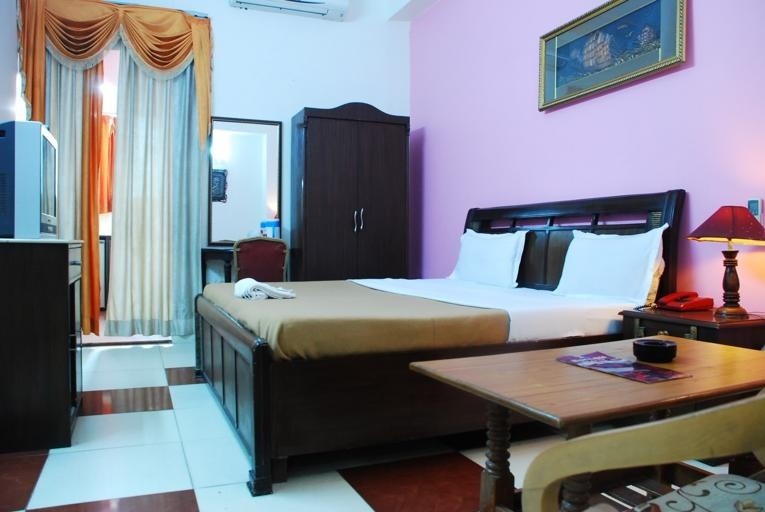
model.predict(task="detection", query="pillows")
[446,227,530,289]
[553,223,670,306]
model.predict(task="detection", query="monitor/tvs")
[0,120,59,240]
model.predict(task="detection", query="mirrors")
[209,116,282,246]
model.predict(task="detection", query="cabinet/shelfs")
[0,238,84,454]
[292,102,410,283]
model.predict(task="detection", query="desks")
[201,248,288,294]
[408,335,765,512]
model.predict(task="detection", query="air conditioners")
[229,0,347,22]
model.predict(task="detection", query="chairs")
[233,237,289,283]
[522,387,765,511]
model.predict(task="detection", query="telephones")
[658,290,714,312]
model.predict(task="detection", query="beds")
[195,189,686,497]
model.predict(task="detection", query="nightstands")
[618,308,765,348]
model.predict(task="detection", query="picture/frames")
[538,1,686,112]
[212,169,228,201]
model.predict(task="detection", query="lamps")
[686,205,765,318]
